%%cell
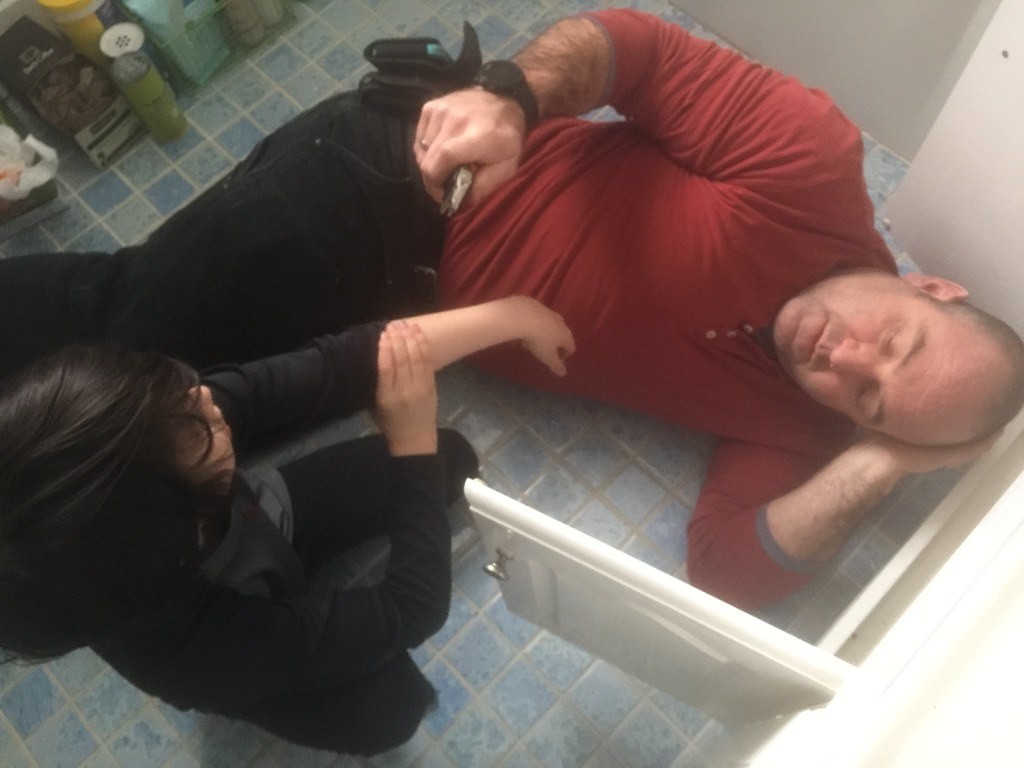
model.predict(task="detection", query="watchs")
[474,59,541,138]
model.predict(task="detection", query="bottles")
[110,52,186,146]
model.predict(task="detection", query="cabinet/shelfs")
[463,400,1022,768]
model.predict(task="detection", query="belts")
[404,118,442,313]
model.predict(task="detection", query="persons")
[2,9,1023,615]
[0,293,577,761]
